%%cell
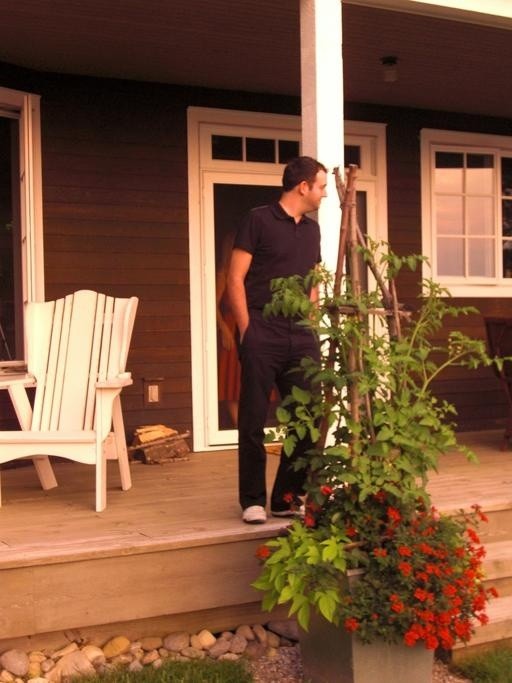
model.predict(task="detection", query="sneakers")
[273,504,307,517]
[242,506,268,524]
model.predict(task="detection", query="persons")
[227,156,328,523]
[217,215,278,428]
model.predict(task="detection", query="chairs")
[0,290,140,513]
